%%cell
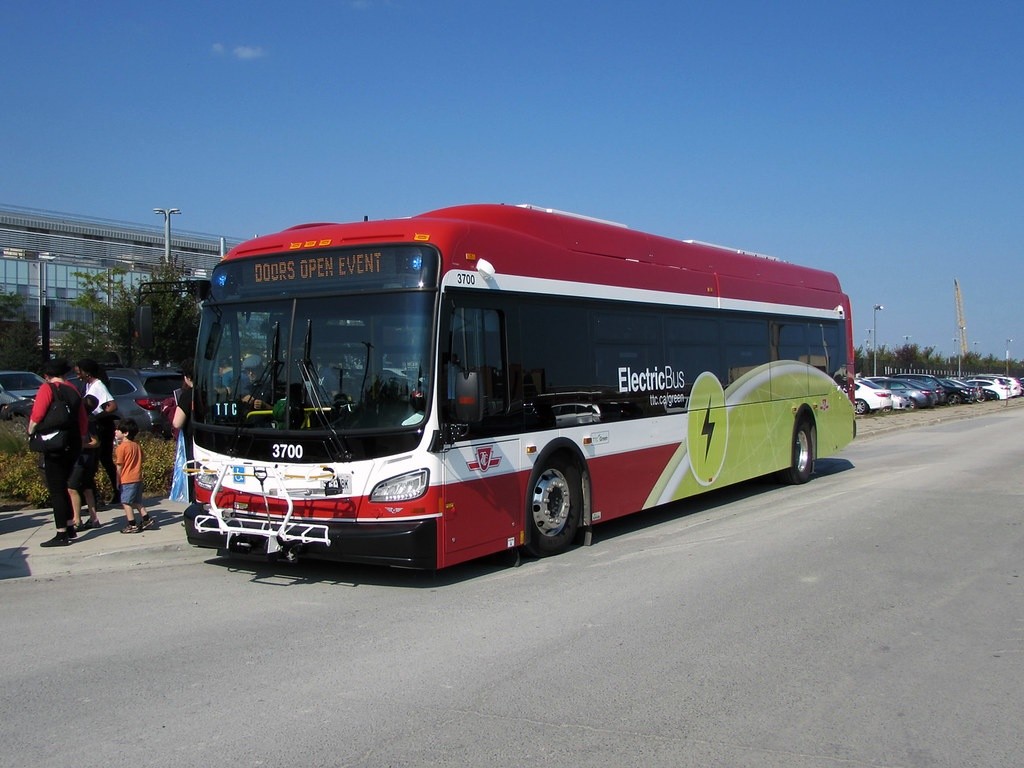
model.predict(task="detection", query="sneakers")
[121,524,139,534]
[137,519,155,531]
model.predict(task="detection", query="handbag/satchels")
[29,383,70,452]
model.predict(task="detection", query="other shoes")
[67,526,76,538]
[84,519,102,529]
[72,522,85,531]
[87,501,106,511]
[40,531,69,546]
[108,492,122,508]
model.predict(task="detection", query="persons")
[28,360,102,547]
[75,359,122,506]
[170,360,195,527]
[114,417,156,533]
[215,354,340,412]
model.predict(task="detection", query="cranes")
[952,275,969,355]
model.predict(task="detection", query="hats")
[244,355,263,372]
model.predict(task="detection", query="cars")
[0,371,47,408]
[854,373,1024,415]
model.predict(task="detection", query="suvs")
[0,368,188,443]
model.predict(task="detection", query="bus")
[181,201,855,572]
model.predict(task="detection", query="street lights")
[903,335,912,350]
[865,328,874,348]
[152,207,182,264]
[953,337,959,358]
[973,341,979,355]
[1004,338,1013,377]
